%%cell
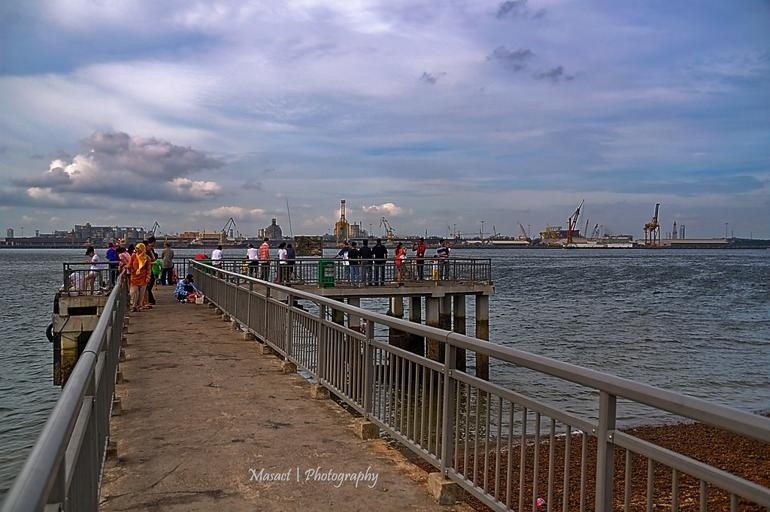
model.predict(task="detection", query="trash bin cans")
[317,261,336,287]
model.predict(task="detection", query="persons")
[211,245,224,276]
[394,237,427,283]
[430,255,441,281]
[259,237,271,282]
[332,241,352,284]
[358,239,373,286]
[82,236,178,312]
[369,237,388,287]
[240,258,250,283]
[277,242,288,283]
[436,239,450,281]
[286,243,296,283]
[347,242,362,287]
[172,273,204,303]
[245,243,260,278]
[444,242,452,263]
